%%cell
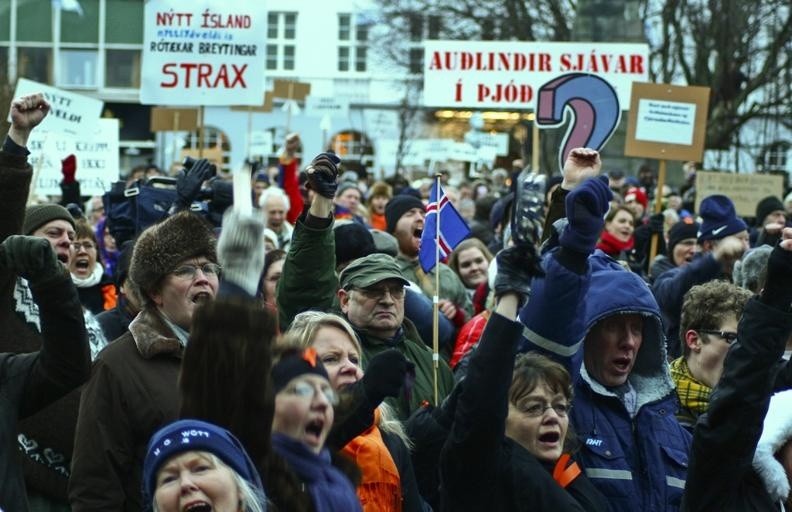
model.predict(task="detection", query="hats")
[335,223,398,264]
[385,195,426,232]
[472,178,512,226]
[339,252,411,291]
[612,167,648,207]
[19,203,75,236]
[140,416,268,510]
[337,181,395,200]
[755,194,785,227]
[697,194,749,242]
[668,220,700,255]
[115,243,134,289]
[128,211,217,295]
[269,350,330,392]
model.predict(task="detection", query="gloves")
[62,154,76,182]
[175,157,211,204]
[304,150,340,198]
[494,247,542,309]
[560,175,613,255]
[2,235,64,286]
[647,213,665,232]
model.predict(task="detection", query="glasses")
[527,400,573,417]
[367,285,406,299]
[698,328,738,344]
[161,263,219,279]
[293,382,340,405]
